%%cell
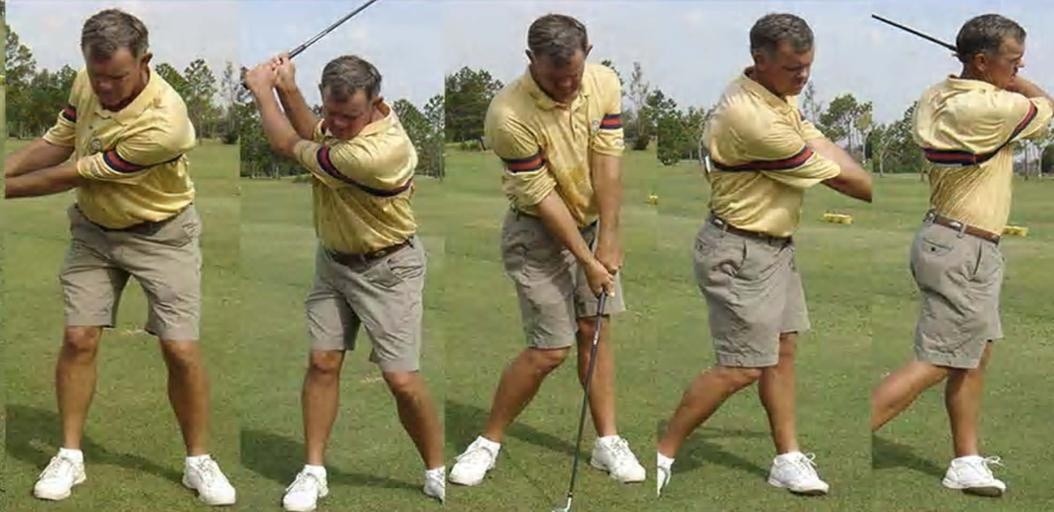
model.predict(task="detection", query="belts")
[328,238,412,266]
[77,203,190,232]
[708,216,792,248]
[927,212,1002,245]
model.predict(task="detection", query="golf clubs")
[551,293,606,512]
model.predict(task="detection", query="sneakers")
[182,458,235,505]
[449,444,500,486]
[590,440,643,483]
[768,455,829,495]
[423,470,445,500]
[283,470,329,512]
[942,457,1005,496]
[657,465,671,499]
[33,457,86,502]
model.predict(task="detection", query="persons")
[3,10,237,506]
[870,15,1054,496]
[657,13,872,495]
[245,54,446,512]
[446,16,646,483]
